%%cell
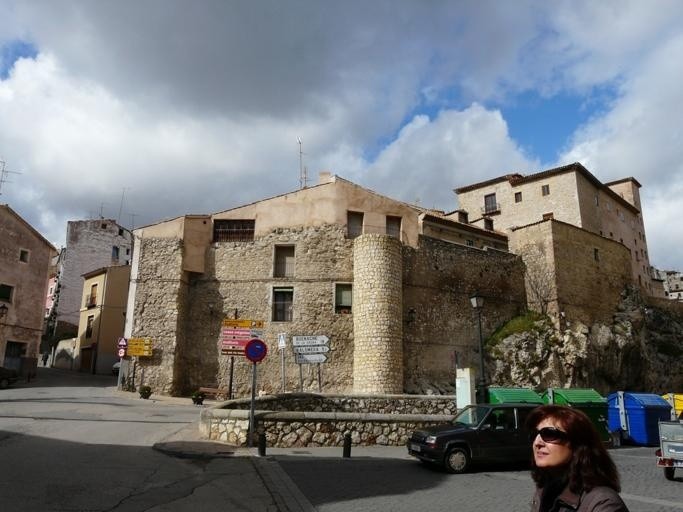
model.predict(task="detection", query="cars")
[0,303,9,319]
[0,368,16,389]
[111,357,120,376]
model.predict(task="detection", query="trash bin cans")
[475,387,683,447]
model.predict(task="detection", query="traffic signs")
[222,338,250,348]
[222,329,263,336]
[291,344,331,354]
[220,349,245,357]
[294,334,329,347]
[297,354,328,364]
[223,319,264,328]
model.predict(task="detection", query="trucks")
[655,408,683,483]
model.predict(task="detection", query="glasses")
[528,427,566,442]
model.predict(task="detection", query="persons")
[42,351,48,367]
[522,406,629,512]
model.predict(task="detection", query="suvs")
[408,402,544,481]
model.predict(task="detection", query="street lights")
[467,287,493,405]
